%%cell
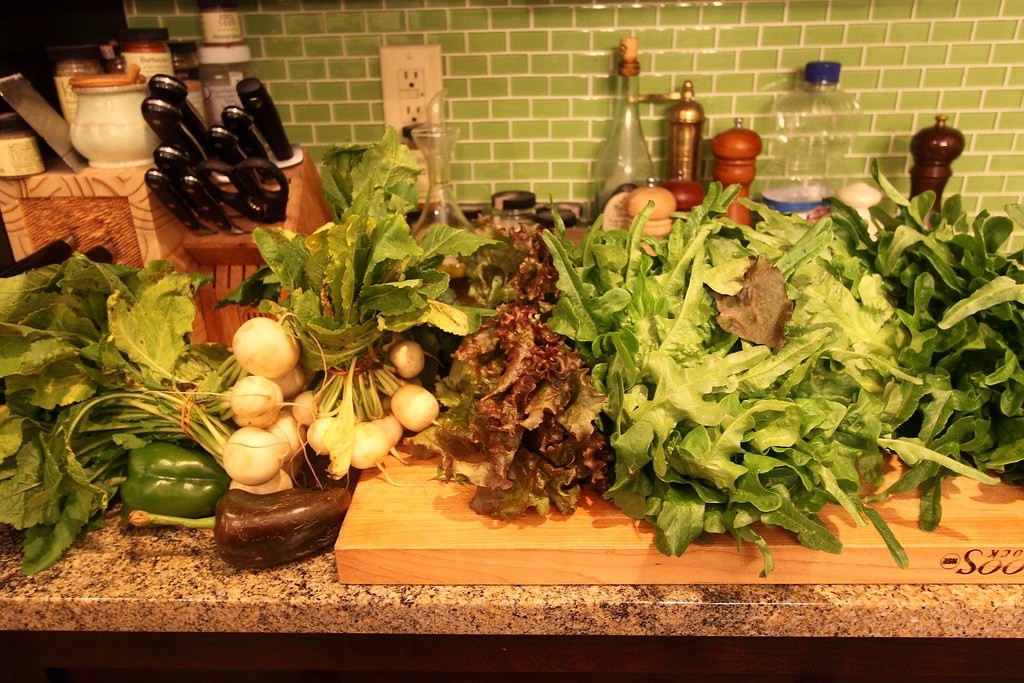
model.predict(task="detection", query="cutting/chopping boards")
[334,434,1024,582]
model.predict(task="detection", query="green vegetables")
[541,158,1023,579]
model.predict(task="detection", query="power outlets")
[375,41,449,154]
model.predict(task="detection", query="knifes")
[209,126,244,163]
[144,169,219,235]
[221,104,291,185]
[140,96,207,162]
[235,78,305,167]
[179,175,252,236]
[154,143,195,179]
[147,73,218,158]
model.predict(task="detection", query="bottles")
[625,176,674,240]
[761,59,861,220]
[840,179,882,241]
[709,115,761,227]
[666,78,706,181]
[906,114,972,236]
[409,125,479,279]
[493,189,536,233]
[167,39,199,69]
[199,41,269,152]
[48,45,104,122]
[0,110,46,181]
[591,35,661,234]
[69,75,164,168]
[118,22,175,85]
[662,169,705,216]
[175,67,206,122]
[538,204,588,248]
[105,53,127,75]
[195,1,244,45]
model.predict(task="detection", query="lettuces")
[400,211,618,524]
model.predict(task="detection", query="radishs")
[0,124,501,576]
